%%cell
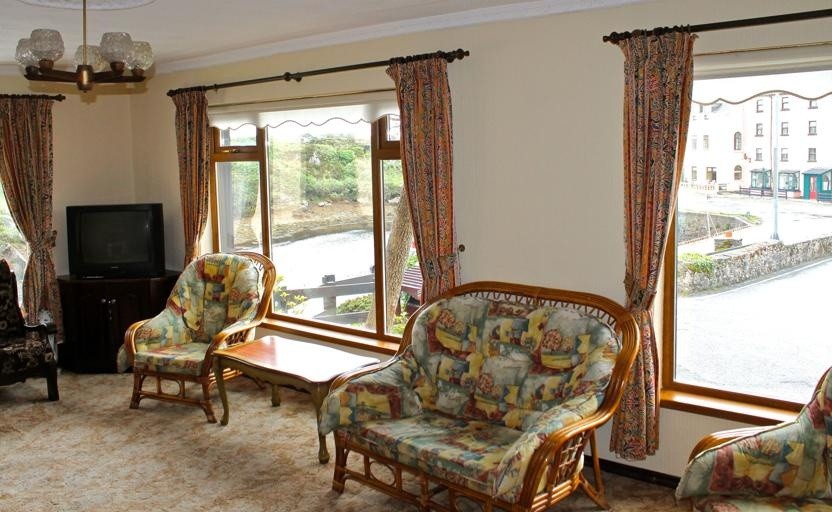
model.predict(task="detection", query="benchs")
[316,280,642,512]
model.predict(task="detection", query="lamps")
[15,0,155,93]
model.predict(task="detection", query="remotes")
[81,275,104,279]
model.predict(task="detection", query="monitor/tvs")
[66,203,166,279]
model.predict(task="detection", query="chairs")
[675,368,831,512]
[120,242,276,425]
[0,256,61,402]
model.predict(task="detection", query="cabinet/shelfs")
[56,269,183,376]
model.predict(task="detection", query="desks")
[209,332,380,465]
